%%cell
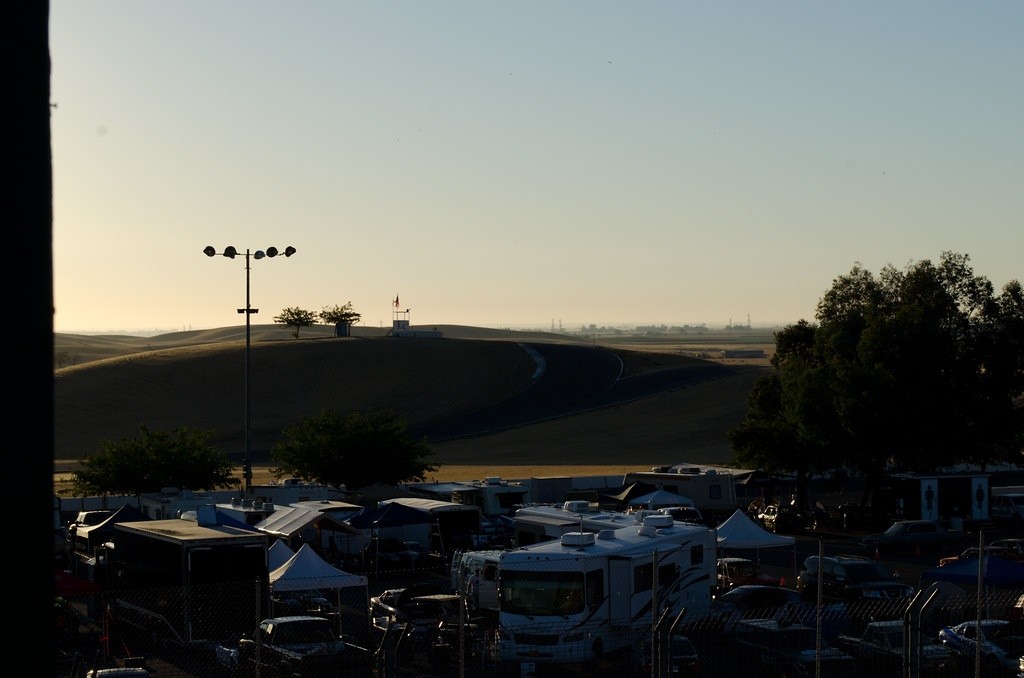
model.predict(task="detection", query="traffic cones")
[915,545,920,557]
[874,548,880,559]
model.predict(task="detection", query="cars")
[657,484,1024,678]
[243,507,519,678]
[63,509,118,556]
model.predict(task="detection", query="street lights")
[204,245,295,496]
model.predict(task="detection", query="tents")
[714,507,796,549]
[628,488,695,508]
[920,554,1024,584]
[344,501,439,530]
[266,538,370,592]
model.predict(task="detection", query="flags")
[395,293,400,308]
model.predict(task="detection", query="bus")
[496,499,718,671]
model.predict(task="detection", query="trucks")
[90,518,269,662]
